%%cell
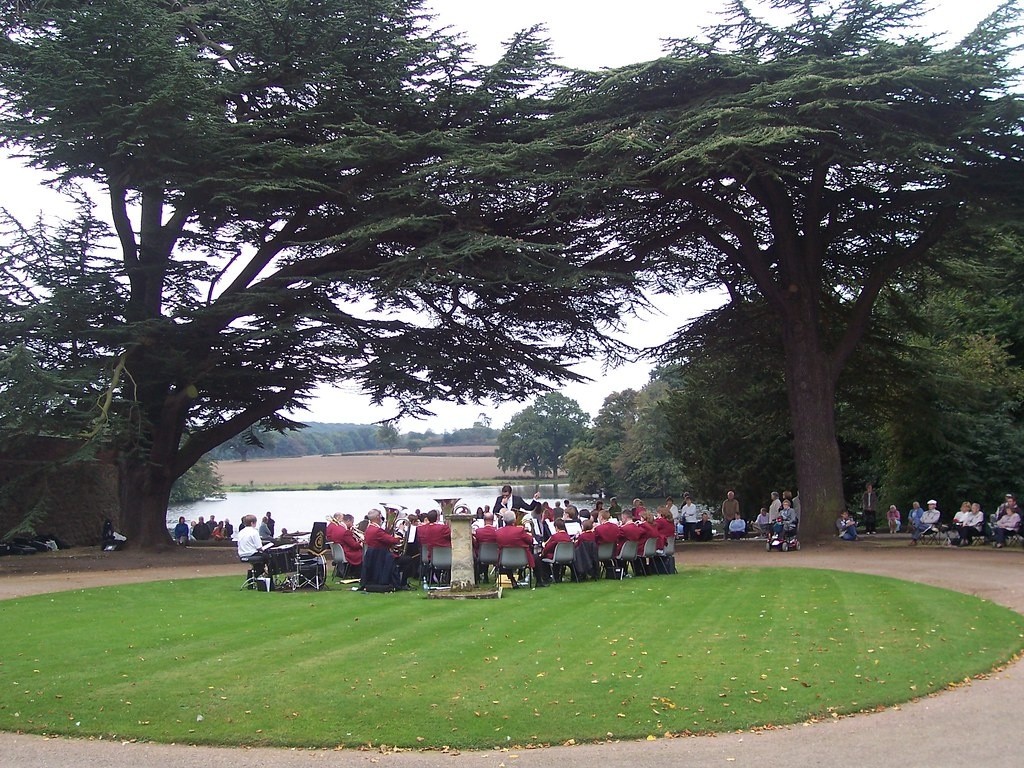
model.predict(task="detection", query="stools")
[240,556,265,591]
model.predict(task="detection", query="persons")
[908,500,940,546]
[175,517,189,545]
[327,509,451,591]
[836,511,864,541]
[953,501,984,547]
[908,502,924,529]
[237,512,275,583]
[102,521,114,550]
[887,505,901,534]
[754,490,801,543]
[681,492,713,542]
[992,493,1024,547]
[280,528,287,537]
[471,485,676,589]
[863,484,877,535]
[729,512,746,541]
[190,515,233,540]
[665,496,679,540]
[722,491,739,541]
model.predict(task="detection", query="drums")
[265,544,299,576]
[294,552,327,589]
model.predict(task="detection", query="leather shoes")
[908,541,917,546]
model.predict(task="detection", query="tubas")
[433,497,471,526]
[379,502,411,558]
[512,508,535,536]
[327,514,367,543]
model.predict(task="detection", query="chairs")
[724,518,749,542]
[326,542,368,581]
[419,536,678,588]
[916,512,1024,548]
[765,517,798,540]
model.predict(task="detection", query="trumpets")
[542,533,552,548]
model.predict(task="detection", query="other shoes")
[958,542,969,547]
[511,577,520,589]
[866,532,876,535]
[536,580,550,588]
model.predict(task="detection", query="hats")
[927,499,937,504]
[1005,493,1013,497]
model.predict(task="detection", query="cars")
[765,531,801,552]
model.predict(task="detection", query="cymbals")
[285,531,312,536]
